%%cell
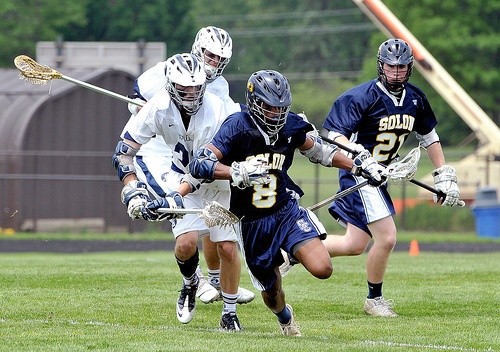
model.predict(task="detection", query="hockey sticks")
[294,112,467,208]
[11,53,145,108]
[308,146,421,213]
[155,201,238,227]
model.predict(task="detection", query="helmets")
[165,53,206,113]
[192,26,233,82]
[377,39,413,92]
[246,69,291,134]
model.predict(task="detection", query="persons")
[111,53,244,334]
[249,38,461,317]
[127,25,256,304]
[188,69,390,338]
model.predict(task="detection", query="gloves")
[431,165,461,207]
[141,192,186,222]
[121,181,152,220]
[351,149,389,188]
[229,155,270,189]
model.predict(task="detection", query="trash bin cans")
[472,204,500,237]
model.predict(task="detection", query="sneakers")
[176,273,199,323]
[220,313,240,330]
[280,249,293,277]
[195,281,219,304]
[278,304,301,336]
[362,296,398,316]
[217,286,255,304]
